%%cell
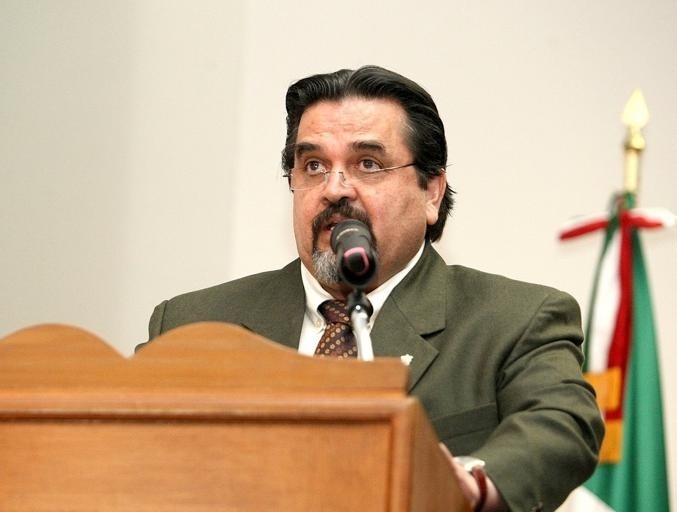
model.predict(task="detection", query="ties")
[313,300,358,358]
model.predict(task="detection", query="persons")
[132,62,608,511]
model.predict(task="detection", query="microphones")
[329,218,379,280]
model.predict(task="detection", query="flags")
[556,193,668,511]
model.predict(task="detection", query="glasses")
[283,162,414,191]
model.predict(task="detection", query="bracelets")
[450,450,489,508]
